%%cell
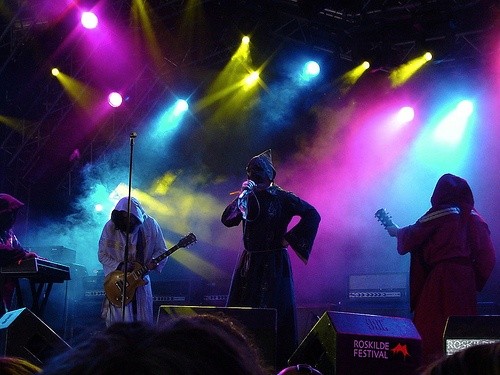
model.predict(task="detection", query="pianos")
[5,255,72,288]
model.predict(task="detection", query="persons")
[97,196,169,328]
[0,192,48,319]
[220,148,322,375]
[0,309,500,375]
[383,172,497,375]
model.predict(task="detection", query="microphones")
[239,181,255,199]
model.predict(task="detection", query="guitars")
[374,208,395,234]
[103,233,197,306]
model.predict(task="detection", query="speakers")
[157,304,278,373]
[287,311,422,375]
[442,315,500,358]
[0,307,73,368]
